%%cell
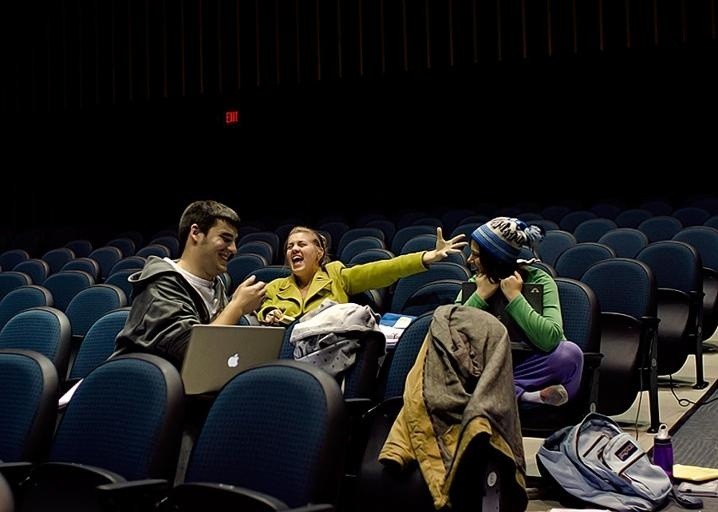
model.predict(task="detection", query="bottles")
[651,423,673,481]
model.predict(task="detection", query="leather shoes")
[541,384,568,406]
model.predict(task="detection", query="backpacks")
[536,412,674,512]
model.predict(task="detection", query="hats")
[471,216,545,261]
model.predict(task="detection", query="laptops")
[180,323,285,394]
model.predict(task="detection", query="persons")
[453,215,584,408]
[104,198,267,365]
[250,224,471,329]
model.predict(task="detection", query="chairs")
[0,208,716,512]
